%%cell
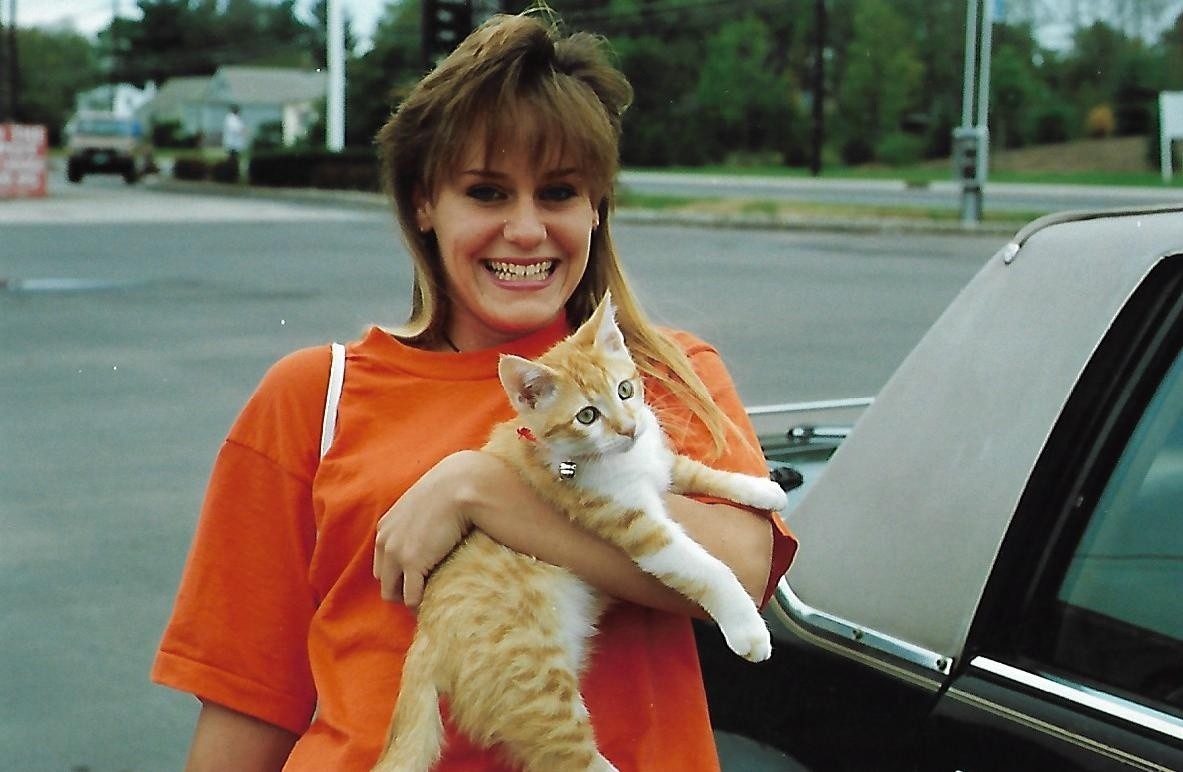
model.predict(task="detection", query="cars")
[692,203,1182,772]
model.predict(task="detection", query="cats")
[368,285,789,772]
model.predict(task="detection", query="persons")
[221,104,250,184]
[151,12,799,772]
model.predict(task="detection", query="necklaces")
[440,328,461,355]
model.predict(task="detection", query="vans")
[68,111,146,184]
